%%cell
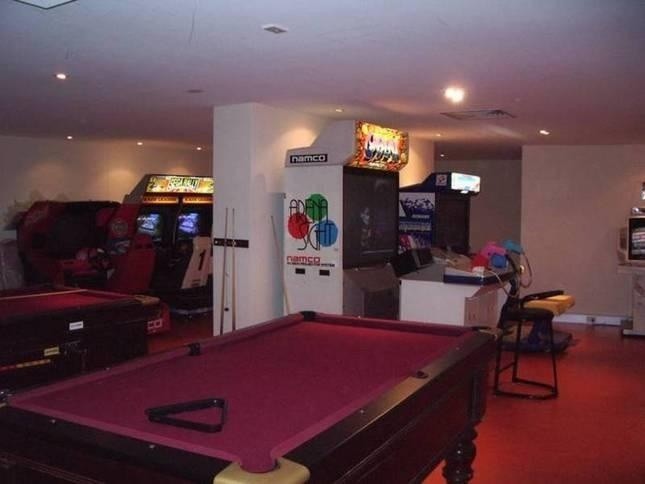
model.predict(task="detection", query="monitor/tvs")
[626,216,645,264]
[176,212,199,241]
[137,213,162,242]
[344,166,399,269]
[436,191,469,255]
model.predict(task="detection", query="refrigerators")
[280,117,410,323]
[399,171,483,278]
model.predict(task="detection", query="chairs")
[162,234,213,323]
[500,291,574,351]
[101,233,157,293]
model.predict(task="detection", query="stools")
[492,310,558,402]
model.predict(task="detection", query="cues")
[270,215,291,316]
[232,208,236,330]
[220,207,228,336]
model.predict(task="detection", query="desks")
[0,289,161,373]
[3,311,503,484]
